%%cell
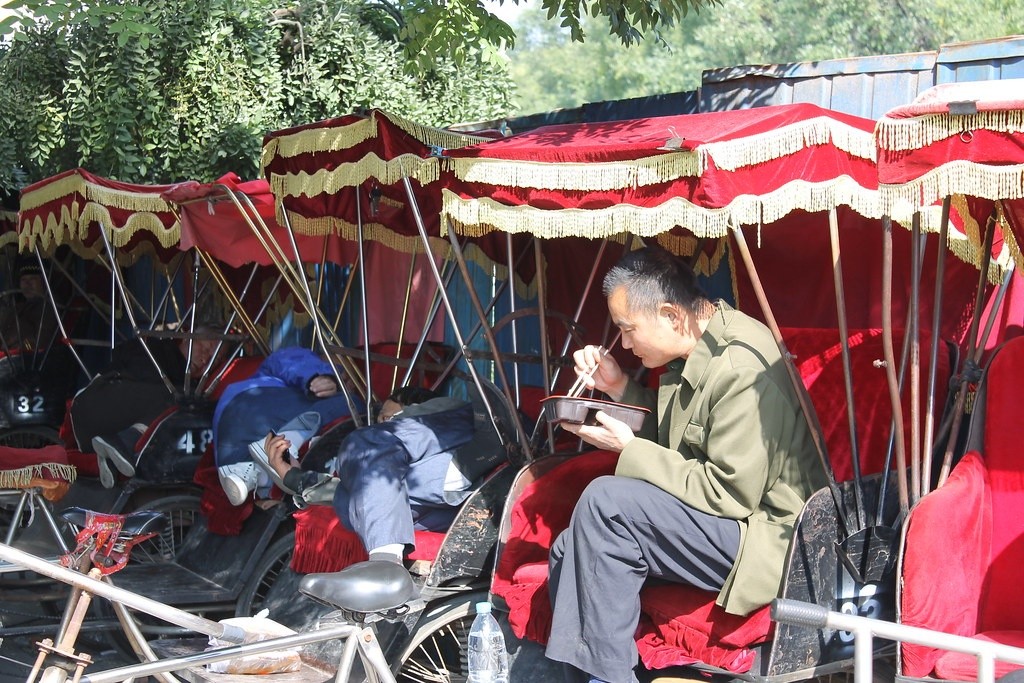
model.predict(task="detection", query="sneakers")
[248,437,296,495]
[218,462,259,506]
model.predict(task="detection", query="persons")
[0,256,63,352]
[69,322,231,488]
[264,387,483,598]
[214,346,364,506]
[545,247,833,683]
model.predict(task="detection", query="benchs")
[197,344,444,507]
[493,328,948,664]
[891,338,1023,683]
[294,384,590,563]
[60,357,263,472]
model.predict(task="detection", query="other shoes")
[95,453,119,489]
[91,433,136,477]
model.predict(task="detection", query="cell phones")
[269,428,292,464]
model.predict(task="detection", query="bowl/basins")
[540,395,652,433]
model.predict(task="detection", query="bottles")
[468,601,508,683]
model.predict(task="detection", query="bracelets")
[306,373,320,394]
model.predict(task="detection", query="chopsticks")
[567,345,608,398]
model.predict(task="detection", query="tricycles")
[0,77,1024,683]
